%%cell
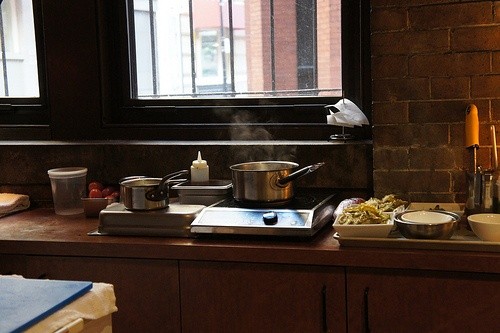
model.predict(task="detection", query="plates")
[404,202,465,218]
[332,212,394,237]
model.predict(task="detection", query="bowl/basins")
[394,209,460,240]
[467,213,500,242]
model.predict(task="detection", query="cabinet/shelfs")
[346,268,500,333]
[22,253,346,333]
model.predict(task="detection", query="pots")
[229,161,328,201]
[120,170,189,210]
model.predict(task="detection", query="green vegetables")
[339,194,407,226]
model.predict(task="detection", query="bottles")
[190,151,209,186]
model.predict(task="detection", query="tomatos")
[88,182,120,199]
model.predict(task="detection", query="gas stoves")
[190,190,344,236]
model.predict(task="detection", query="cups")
[47,167,87,215]
